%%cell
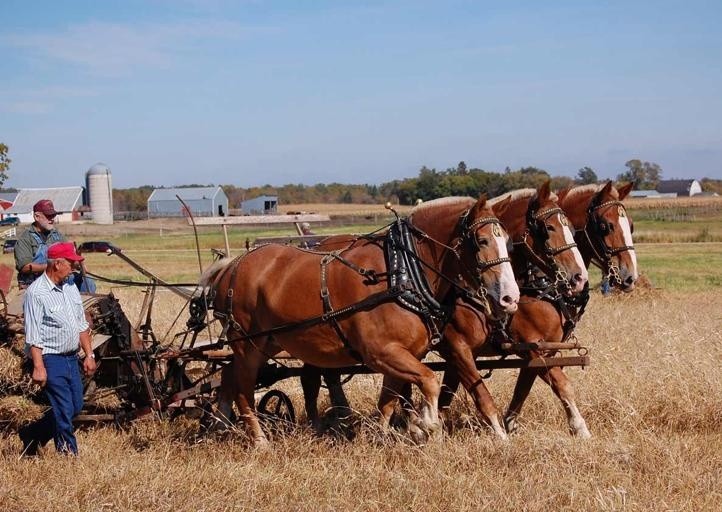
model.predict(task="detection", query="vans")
[77,242,121,253]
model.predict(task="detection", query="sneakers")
[18,424,37,456]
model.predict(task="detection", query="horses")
[194,191,524,450]
[299,178,591,437]
[397,175,641,437]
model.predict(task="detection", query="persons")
[601,213,633,296]
[14,200,97,293]
[22,242,96,456]
[300,222,317,246]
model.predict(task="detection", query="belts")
[61,351,77,357]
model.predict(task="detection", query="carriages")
[1,180,640,455]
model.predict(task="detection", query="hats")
[48,242,85,261]
[33,200,63,216]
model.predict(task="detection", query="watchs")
[86,354,95,358]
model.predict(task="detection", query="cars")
[2,241,17,253]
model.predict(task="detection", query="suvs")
[1,217,19,226]
